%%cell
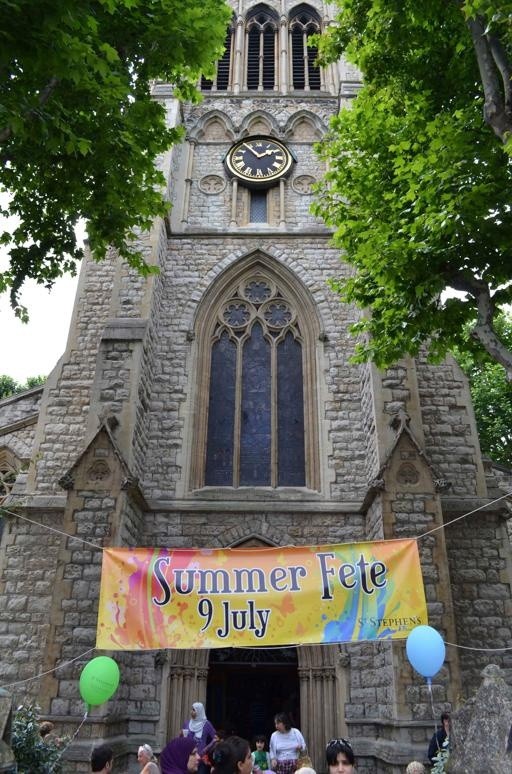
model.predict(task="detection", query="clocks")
[222,131,299,185]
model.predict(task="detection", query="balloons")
[405,624,446,684]
[79,655,120,712]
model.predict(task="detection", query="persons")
[427,712,450,764]
[90,744,114,774]
[406,760,426,774]
[179,702,217,757]
[325,737,355,774]
[37,721,55,746]
[268,712,306,774]
[295,767,317,774]
[136,731,271,774]
